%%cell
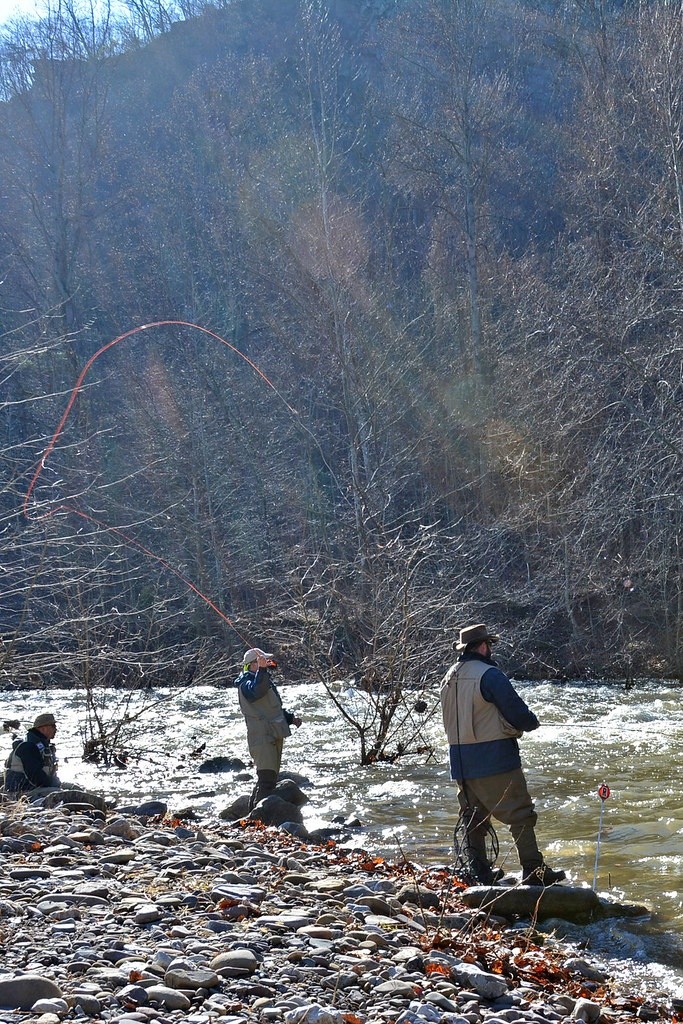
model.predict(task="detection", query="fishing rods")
[539,722,675,737]
[50,501,278,671]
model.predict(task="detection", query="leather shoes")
[522,863,566,886]
[470,865,504,885]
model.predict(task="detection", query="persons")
[9,713,117,810]
[438,622,566,884]
[234,647,303,814]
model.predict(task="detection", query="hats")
[451,622,498,654]
[32,714,57,729]
[242,648,277,669]
